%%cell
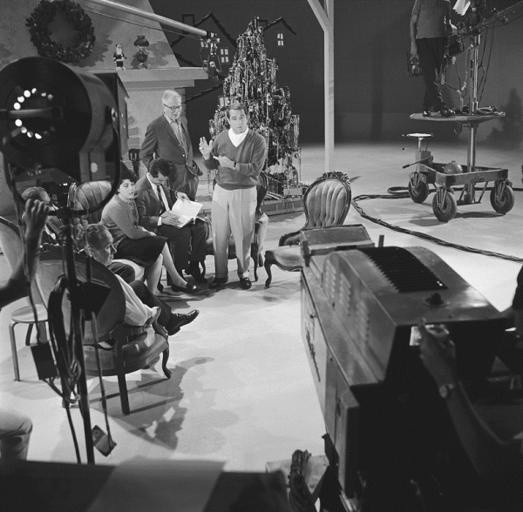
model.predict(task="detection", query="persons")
[74,224,199,346]
[409,0,456,117]
[198,105,266,289]
[134,158,207,291]
[21,187,135,284]
[101,174,198,296]
[141,89,203,202]
[417,266,523,510]
[1,198,49,460]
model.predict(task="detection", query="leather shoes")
[172,264,227,292]
[422,107,456,117]
[165,310,198,335]
[240,277,251,289]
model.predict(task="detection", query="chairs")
[0,216,43,346]
[33,244,171,417]
[264,171,351,288]
[70,181,164,291]
[200,171,269,283]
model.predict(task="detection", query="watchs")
[437,383,457,399]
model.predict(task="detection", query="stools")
[9,303,49,381]
[160,216,210,284]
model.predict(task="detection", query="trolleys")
[400,1,522,223]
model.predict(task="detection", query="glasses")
[163,104,180,110]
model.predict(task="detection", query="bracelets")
[233,161,237,171]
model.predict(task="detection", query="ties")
[157,185,166,212]
